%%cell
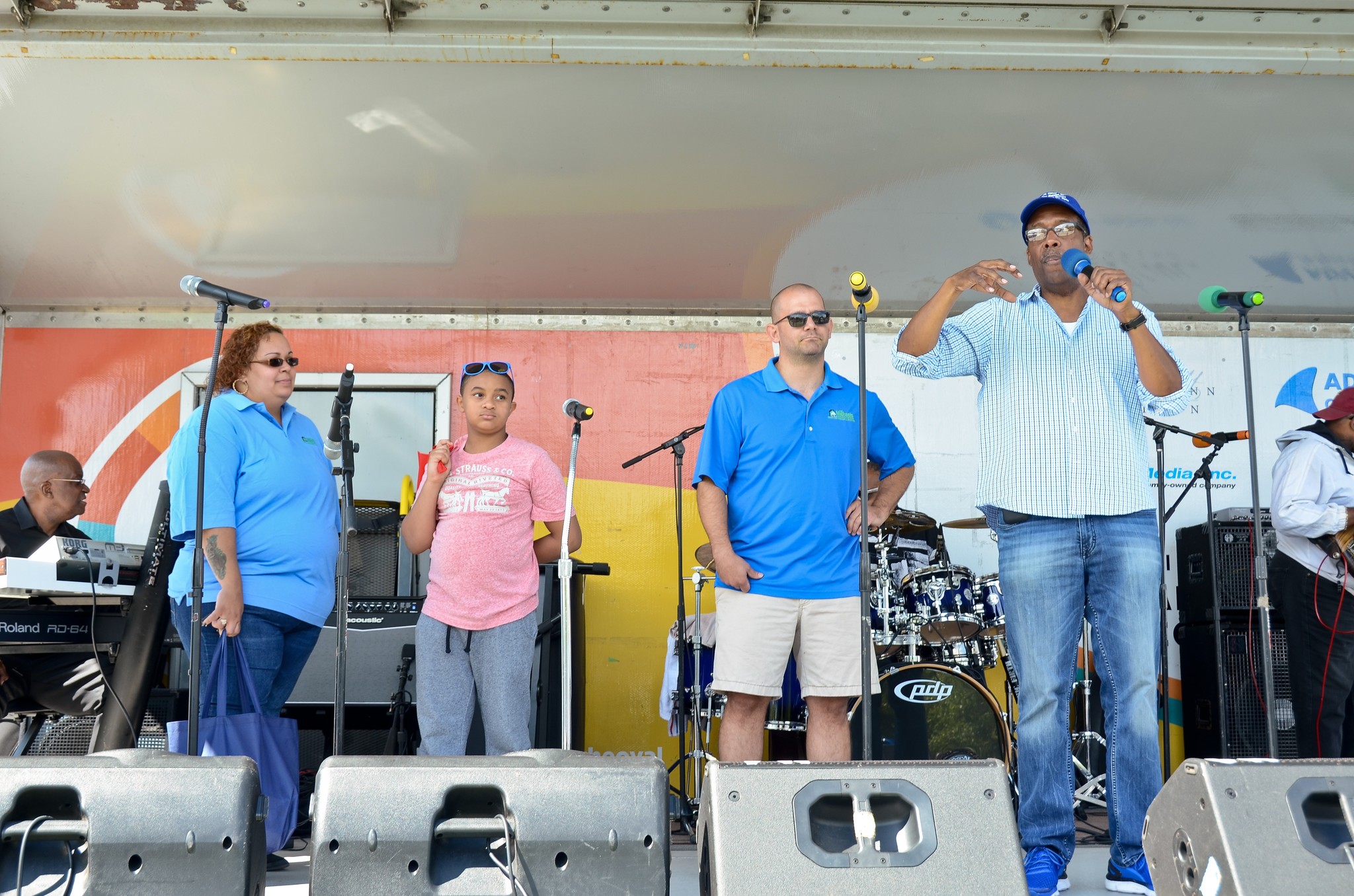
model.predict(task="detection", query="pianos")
[0,535,147,654]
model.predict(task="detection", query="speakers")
[1175,519,1302,765]
[1141,756,1354,896]
[697,756,1029,896]
[0,745,272,895]
[338,499,412,595]
[281,600,425,710]
[309,748,672,895]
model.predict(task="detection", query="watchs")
[1119,309,1147,333]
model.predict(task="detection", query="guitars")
[1334,524,1354,578]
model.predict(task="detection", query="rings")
[220,618,227,625]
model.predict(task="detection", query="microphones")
[401,644,416,663]
[180,275,270,310]
[1192,430,1248,448]
[562,398,594,420]
[1197,286,1264,314]
[1061,248,1126,303]
[849,271,879,313]
[323,363,355,460]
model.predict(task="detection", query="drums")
[975,571,1006,640]
[846,659,1010,777]
[933,636,997,671]
[673,638,723,717]
[763,647,808,731]
[870,569,908,660]
[901,564,981,647]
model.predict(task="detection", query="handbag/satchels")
[167,630,301,856]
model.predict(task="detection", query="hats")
[1313,387,1354,420]
[1021,192,1090,245]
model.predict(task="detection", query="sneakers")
[1104,856,1157,896]
[1022,846,1071,896]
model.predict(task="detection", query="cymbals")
[868,510,937,535]
[942,515,990,528]
[694,542,716,573]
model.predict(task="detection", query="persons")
[402,361,582,758]
[166,321,341,872]
[890,192,1194,896]
[1,451,114,722]
[693,283,915,761]
[1270,387,1353,758]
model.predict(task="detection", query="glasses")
[459,361,514,393]
[1025,223,1087,242]
[774,310,831,328]
[248,358,298,367]
[46,479,85,488]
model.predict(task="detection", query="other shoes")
[264,853,290,871]
[1305,804,1347,826]
